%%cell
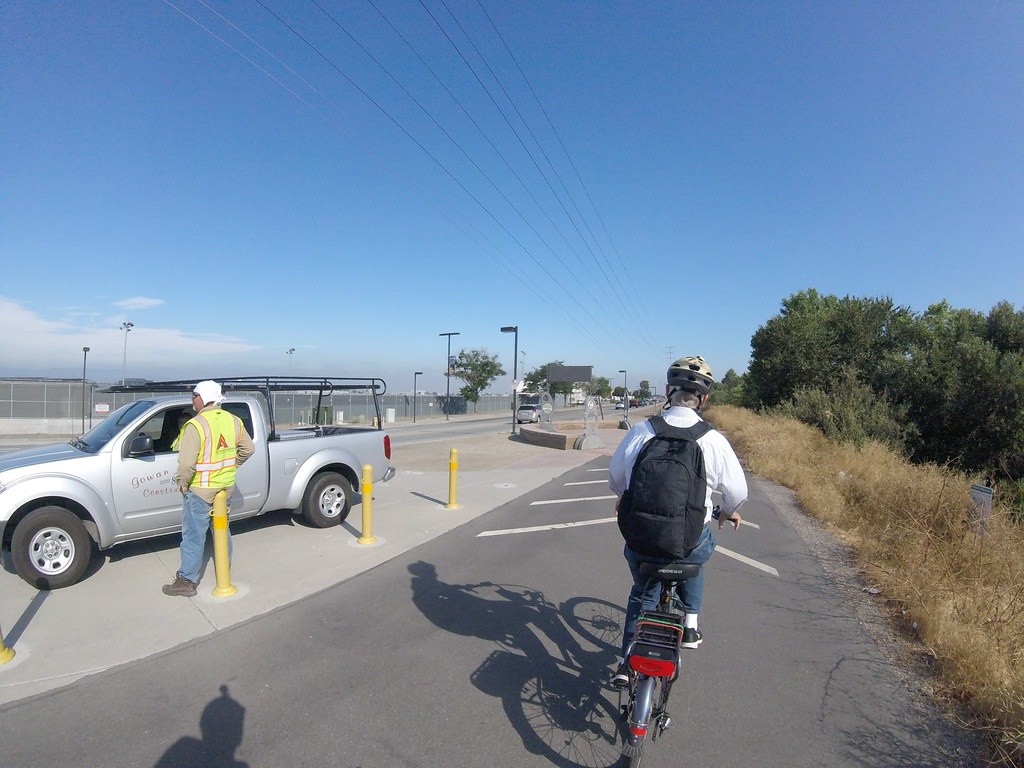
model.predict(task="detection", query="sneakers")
[609,661,630,685]
[162,570,198,597]
[680,626,703,649]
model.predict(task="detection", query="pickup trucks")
[0,375,395,591]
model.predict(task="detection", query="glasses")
[191,390,198,400]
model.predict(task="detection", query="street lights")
[651,386,657,407]
[618,370,629,421]
[500,325,519,434]
[438,333,460,421]
[120,320,135,386]
[286,347,296,376]
[82,346,90,435]
[413,371,424,422]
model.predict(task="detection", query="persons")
[162,380,255,596]
[610,355,749,685]
[171,412,192,450]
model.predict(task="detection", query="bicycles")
[609,503,736,767]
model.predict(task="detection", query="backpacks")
[617,416,716,561]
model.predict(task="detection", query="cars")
[517,404,550,424]
[577,400,584,406]
[615,398,664,410]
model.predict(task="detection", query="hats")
[193,380,227,405]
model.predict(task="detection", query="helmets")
[666,355,715,394]
